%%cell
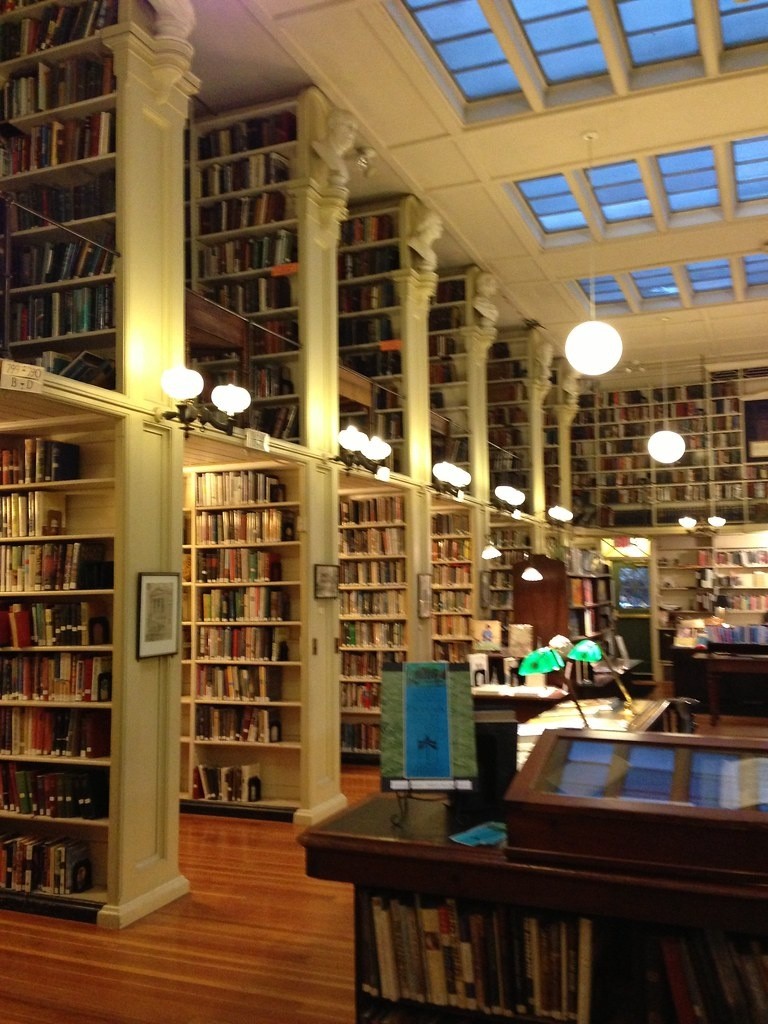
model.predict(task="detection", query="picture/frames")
[136,571,181,662]
[314,562,340,601]
[418,573,432,618]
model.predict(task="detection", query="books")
[0,1,767,1023]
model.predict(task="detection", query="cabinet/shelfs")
[0,0,768,522]
[297,790,768,1024]
[0,405,768,929]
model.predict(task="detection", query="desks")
[557,655,645,704]
[601,506,615,527]
[692,650,768,724]
[339,364,378,455]
[472,684,569,725]
[431,410,451,462]
[184,289,254,427]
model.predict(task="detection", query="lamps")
[156,238,731,560]
[568,637,637,716]
[518,645,594,728]
